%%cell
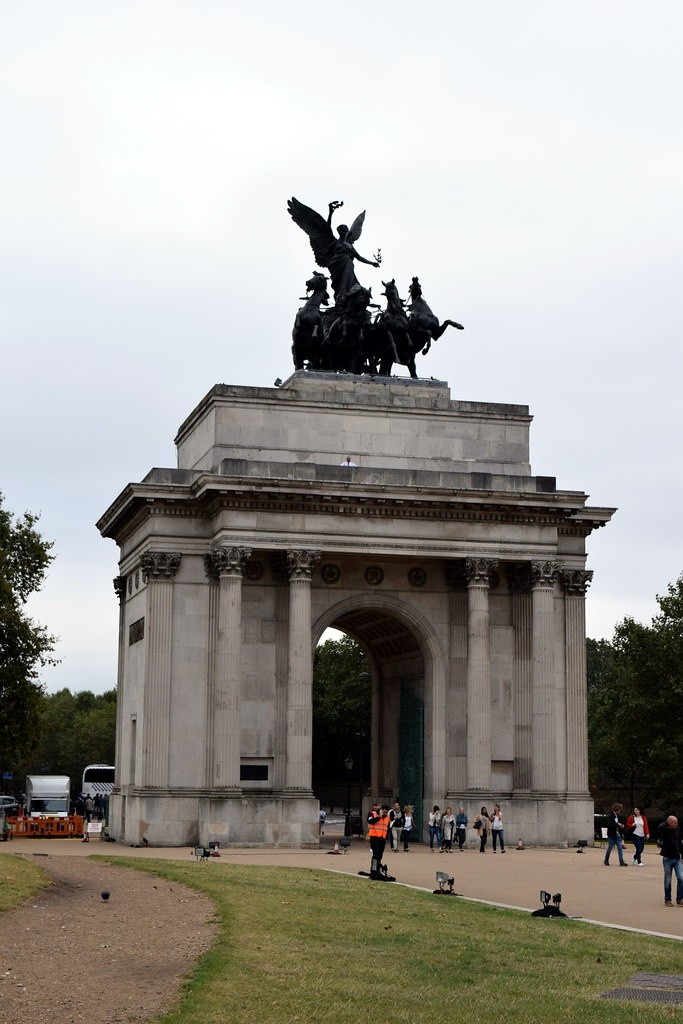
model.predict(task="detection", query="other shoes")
[460,848,464,852]
[394,846,399,853]
[450,849,453,853]
[632,855,636,865]
[677,898,683,904]
[440,849,442,853]
[431,848,434,853]
[494,850,496,853]
[502,850,505,853]
[620,862,628,866]
[445,849,449,852]
[665,899,674,907]
[403,848,409,852]
[480,849,485,852]
[637,862,644,866]
[604,859,609,866]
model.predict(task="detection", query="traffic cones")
[332,841,341,855]
[516,837,525,850]
[212,844,221,857]
[622,837,626,849]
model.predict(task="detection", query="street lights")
[344,756,353,818]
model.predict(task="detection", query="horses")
[291,271,465,379]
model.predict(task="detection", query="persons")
[366,801,506,877]
[0,788,25,821]
[323,225,381,304]
[602,803,650,867]
[319,807,325,836]
[3,815,11,840]
[657,814,683,908]
[70,791,109,825]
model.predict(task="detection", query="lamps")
[203,848,211,862]
[209,842,220,849]
[539,891,551,908]
[340,839,351,855]
[371,857,388,876]
[448,876,454,896]
[577,840,587,853]
[553,893,561,910]
[195,846,203,861]
[435,871,449,894]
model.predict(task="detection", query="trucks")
[26,774,72,836]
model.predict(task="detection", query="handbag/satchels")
[626,815,636,834]
[473,820,482,829]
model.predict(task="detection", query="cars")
[0,796,19,818]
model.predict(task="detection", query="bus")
[81,764,115,797]
